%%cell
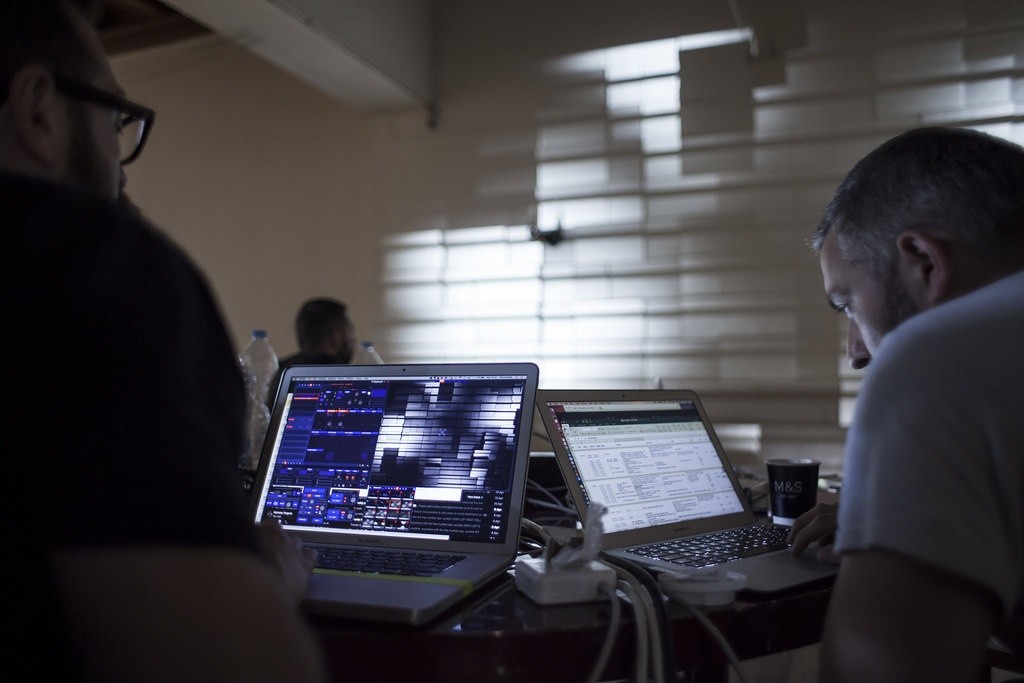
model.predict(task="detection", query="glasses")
[0,72,155,166]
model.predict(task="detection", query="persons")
[0,2,332,682]
[784,129,1024,683]
[264,297,357,421]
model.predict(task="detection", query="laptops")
[537,388,840,598]
[244,363,540,626]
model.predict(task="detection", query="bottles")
[353,341,384,365]
[237,329,279,470]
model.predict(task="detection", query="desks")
[300,460,839,683]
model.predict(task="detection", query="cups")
[764,455,821,526]
